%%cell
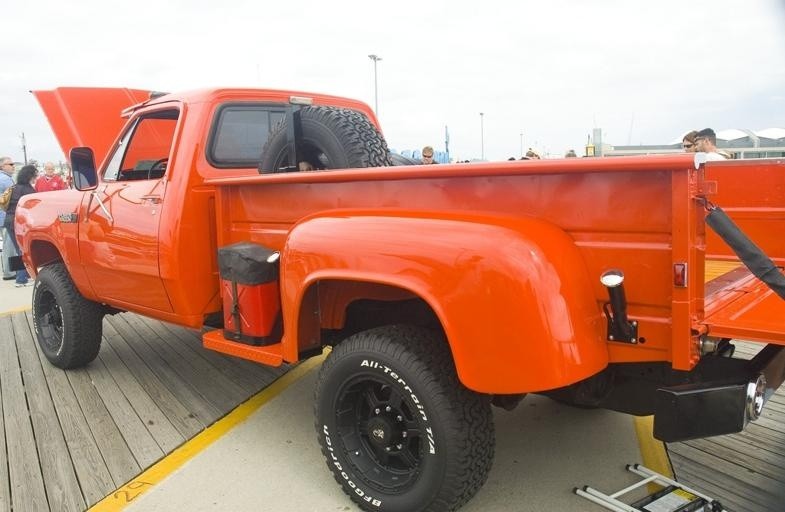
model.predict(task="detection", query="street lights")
[369,54,384,115]
[519,134,524,158]
[478,112,486,161]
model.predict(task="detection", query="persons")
[3,164,38,289]
[0,156,16,280]
[694,128,733,159]
[33,162,68,193]
[420,146,437,164]
[682,131,699,153]
[453,149,594,166]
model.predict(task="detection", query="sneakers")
[15,279,35,287]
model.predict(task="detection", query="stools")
[572,463,727,512]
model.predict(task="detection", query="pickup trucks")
[7,81,785,511]
